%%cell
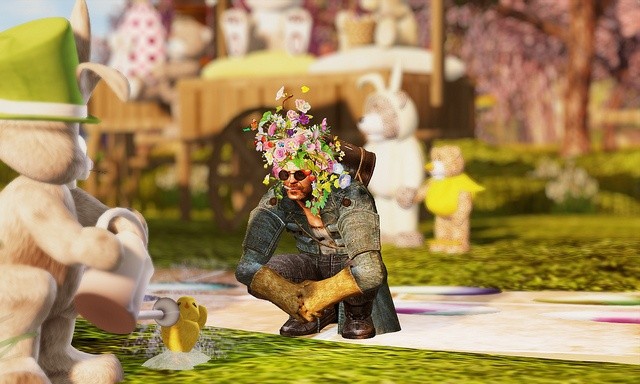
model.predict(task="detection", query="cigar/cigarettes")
[279,183,293,192]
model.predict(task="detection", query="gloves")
[247,266,322,322]
[296,264,366,316]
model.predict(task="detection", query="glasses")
[278,169,305,182]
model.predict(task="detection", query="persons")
[235,160,401,339]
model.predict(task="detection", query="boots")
[279,304,337,336]
[342,300,376,340]
